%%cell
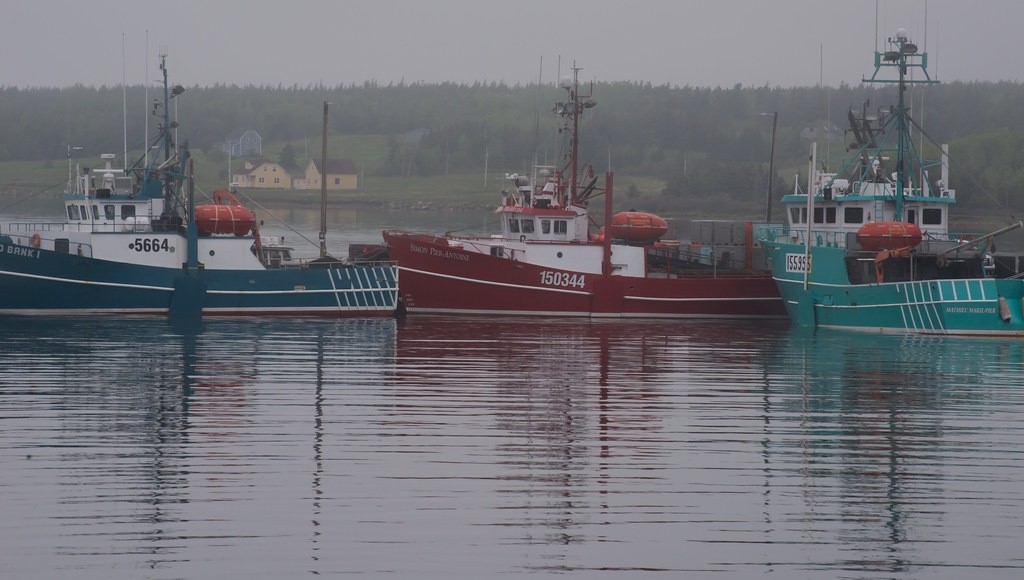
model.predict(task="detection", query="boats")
[380,53,790,321]
[0,32,407,317]
[766,0,1024,337]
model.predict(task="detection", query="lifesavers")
[31,233,41,248]
